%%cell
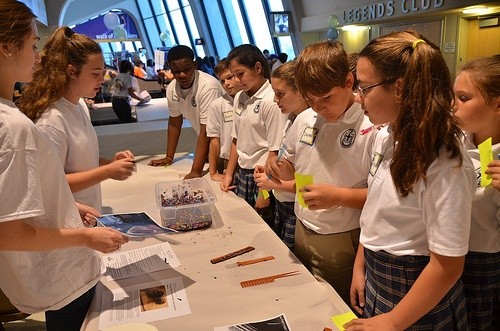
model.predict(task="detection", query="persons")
[0,0,127,331]
[32,26,500,331]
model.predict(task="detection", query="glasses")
[358,79,397,96]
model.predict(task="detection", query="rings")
[118,245,121,250]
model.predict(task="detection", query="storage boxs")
[155,178,217,231]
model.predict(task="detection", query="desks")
[80,98,358,331]
